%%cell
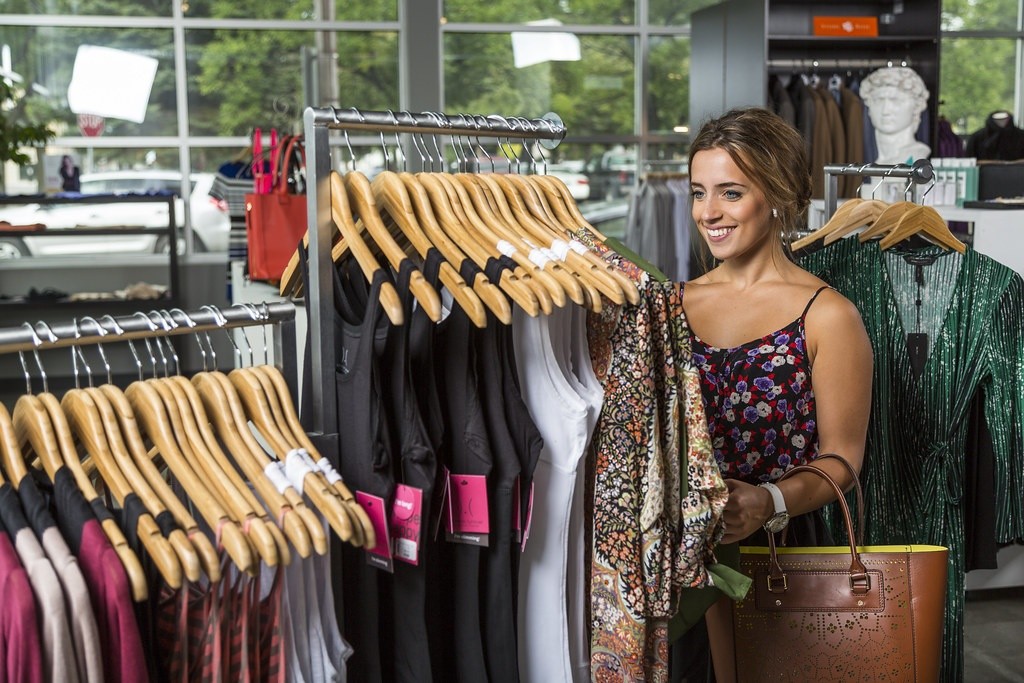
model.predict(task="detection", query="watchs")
[757,479,790,533]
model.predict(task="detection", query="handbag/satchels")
[704,454,948,682]
[244,135,308,281]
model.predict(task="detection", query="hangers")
[268,104,648,330]
[766,55,927,93]
[0,294,376,615]
[782,156,975,259]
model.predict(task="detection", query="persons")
[668,105,873,682]
[857,67,930,172]
[57,154,80,194]
